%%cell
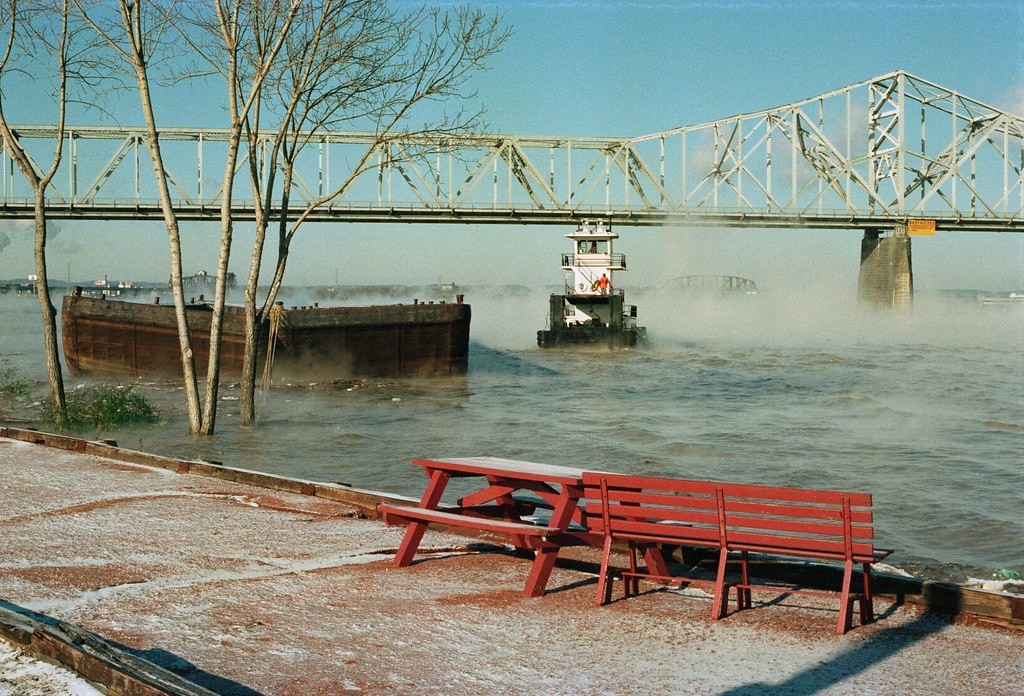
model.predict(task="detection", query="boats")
[537,227,648,349]
[61,286,472,385]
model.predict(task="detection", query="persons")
[597,274,613,293]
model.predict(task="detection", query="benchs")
[582,470,893,636]
[377,503,590,549]
[513,495,699,553]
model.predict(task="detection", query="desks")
[393,456,672,600]
